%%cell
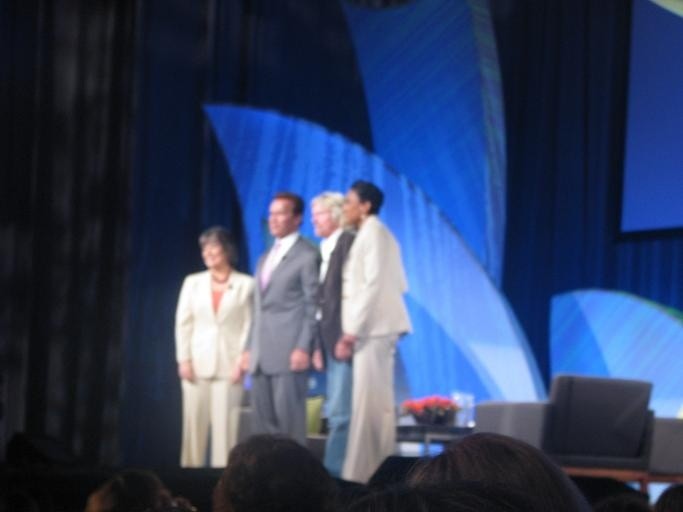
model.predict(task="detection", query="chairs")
[539,374,653,501]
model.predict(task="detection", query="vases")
[416,414,447,432]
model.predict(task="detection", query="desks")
[394,426,473,460]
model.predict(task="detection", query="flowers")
[398,398,461,417]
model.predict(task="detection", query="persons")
[406,431,593,512]
[594,493,651,511]
[306,190,356,477]
[208,434,329,512]
[83,468,197,512]
[241,192,322,458]
[172,225,258,471]
[655,483,683,512]
[344,484,515,511]
[335,177,415,484]
